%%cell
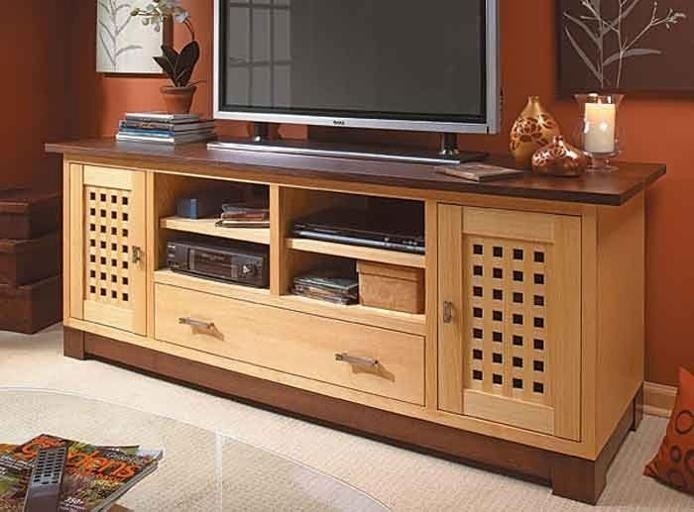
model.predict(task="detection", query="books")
[214,201,271,228]
[0,433,164,512]
[434,163,524,182]
[291,264,359,305]
[115,111,217,146]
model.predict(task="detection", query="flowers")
[129,0,199,87]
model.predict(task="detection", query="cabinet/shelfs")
[43,135,148,371]
[439,144,667,506]
[147,134,438,454]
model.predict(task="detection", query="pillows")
[641,364,692,496]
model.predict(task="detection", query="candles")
[581,98,617,153]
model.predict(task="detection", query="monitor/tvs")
[206,0,502,166]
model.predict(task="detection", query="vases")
[506,93,589,178]
[159,85,196,115]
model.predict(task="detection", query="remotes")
[22,447,68,512]
